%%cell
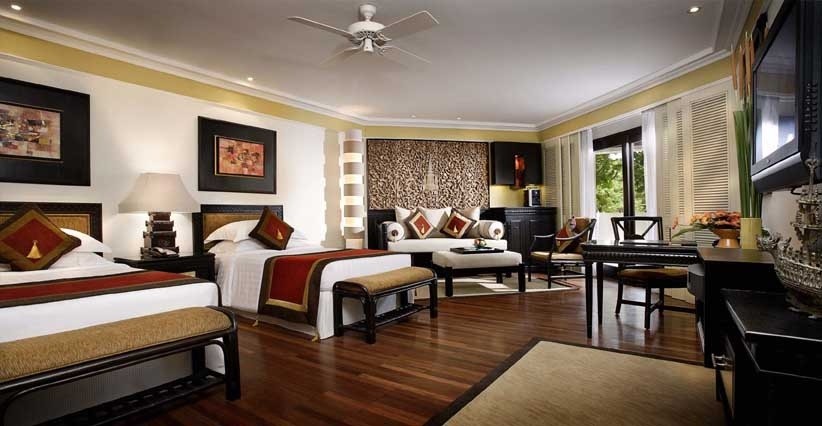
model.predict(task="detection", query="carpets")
[413,269,584,300]
[422,336,729,425]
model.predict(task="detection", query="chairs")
[527,216,595,289]
[610,216,690,330]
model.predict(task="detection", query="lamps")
[117,171,200,256]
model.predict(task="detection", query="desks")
[580,238,698,338]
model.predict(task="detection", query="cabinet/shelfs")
[685,245,777,369]
[709,286,822,426]
[488,207,556,272]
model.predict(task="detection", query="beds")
[191,202,411,342]
[1,202,243,425]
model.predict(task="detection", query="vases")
[710,228,741,248]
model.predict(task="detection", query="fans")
[286,5,440,74]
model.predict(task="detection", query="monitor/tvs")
[751,0,822,192]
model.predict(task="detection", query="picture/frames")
[0,76,92,187]
[196,115,277,195]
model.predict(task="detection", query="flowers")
[668,210,741,240]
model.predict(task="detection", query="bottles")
[478,238,486,247]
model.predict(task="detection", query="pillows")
[203,219,260,242]
[380,203,481,241]
[0,202,81,272]
[59,228,112,254]
[554,215,580,253]
[248,205,294,250]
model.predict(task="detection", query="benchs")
[368,210,507,265]
[431,249,526,295]
[0,305,242,426]
[332,267,438,343]
